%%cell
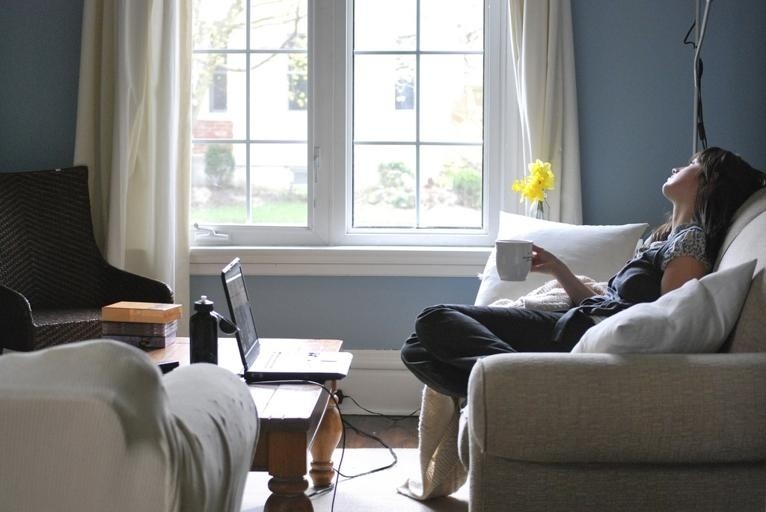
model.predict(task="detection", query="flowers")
[511,159,556,202]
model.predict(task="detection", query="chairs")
[418,184,765,509]
[1,340,259,511]
[0,166,173,346]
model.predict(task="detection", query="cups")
[495,240,533,281]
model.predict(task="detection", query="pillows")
[572,256,758,352]
[472,210,647,309]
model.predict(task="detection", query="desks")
[136,335,343,511]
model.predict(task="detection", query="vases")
[536,202,545,219]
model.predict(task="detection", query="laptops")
[220,256,354,380]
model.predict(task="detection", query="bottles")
[190,295,237,364]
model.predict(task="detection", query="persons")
[400,145,765,398]
